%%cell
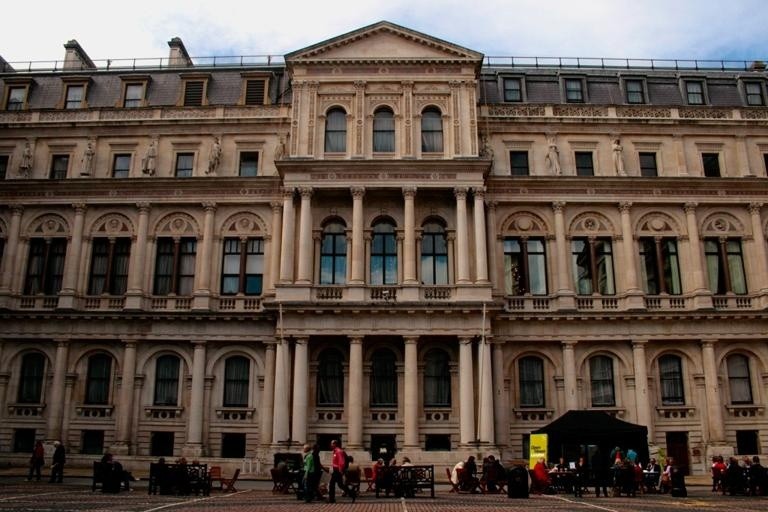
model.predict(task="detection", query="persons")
[707,455,768,494]
[151,457,212,493]
[296,441,416,506]
[103,452,141,492]
[23,439,45,482]
[446,443,687,499]
[48,440,66,483]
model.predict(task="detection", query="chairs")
[148,461,240,497]
[92,461,129,494]
[270,465,434,498]
[447,470,680,494]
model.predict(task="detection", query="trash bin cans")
[506,464,529,498]
[670,466,687,498]
